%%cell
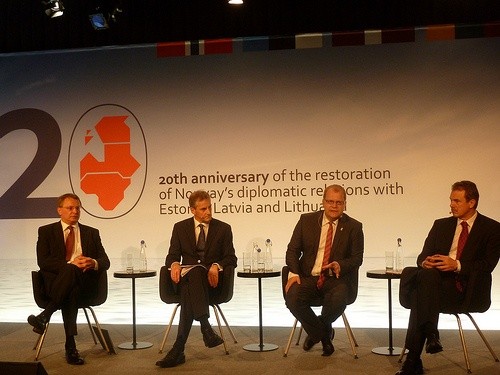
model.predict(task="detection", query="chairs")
[157,266,237,355]
[399,274,499,374]
[32,270,112,361]
[281,266,359,361]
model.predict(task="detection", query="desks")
[367,269,409,356]
[114,269,157,350]
[238,271,281,352]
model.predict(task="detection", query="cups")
[242,251,251,273]
[125,254,133,271]
[385,251,394,271]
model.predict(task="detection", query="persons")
[26,193,110,365]
[155,191,237,368]
[284,184,364,356]
[395,180,500,375]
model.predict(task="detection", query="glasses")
[63,206,80,212]
[323,198,346,205]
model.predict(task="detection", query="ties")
[197,224,205,261]
[317,221,334,290]
[65,225,75,262]
[454,222,469,291]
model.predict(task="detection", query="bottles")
[253,239,272,271]
[395,238,403,272]
[139,241,147,272]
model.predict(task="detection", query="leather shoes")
[321,328,335,355]
[426,329,444,354]
[392,358,423,375]
[303,336,315,351]
[65,341,85,365]
[203,332,224,348]
[155,350,185,367]
[27,315,48,335]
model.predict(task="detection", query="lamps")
[43,0,66,18]
[88,0,123,30]
